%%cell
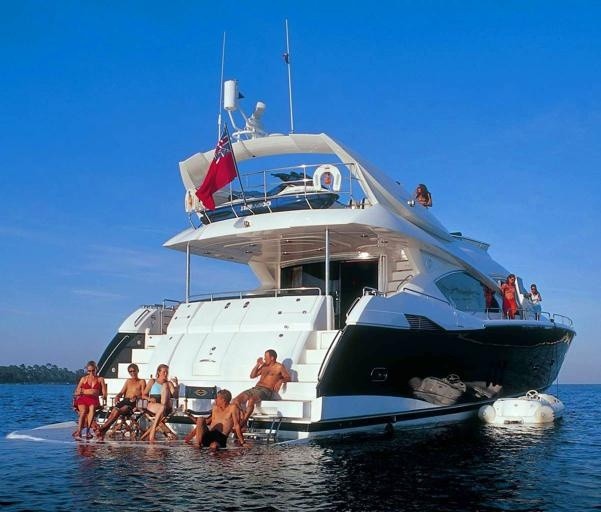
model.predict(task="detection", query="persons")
[227,348,290,431]
[71,360,107,438]
[413,184,431,208]
[92,363,147,438]
[194,388,255,450]
[483,285,494,308]
[500,273,519,320]
[525,285,542,321]
[140,364,178,440]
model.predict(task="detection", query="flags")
[193,129,237,211]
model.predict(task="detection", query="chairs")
[73,384,283,445]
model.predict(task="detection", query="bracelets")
[241,442,245,445]
